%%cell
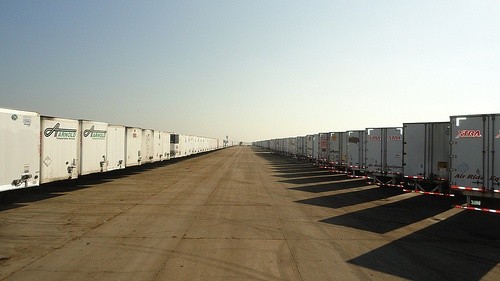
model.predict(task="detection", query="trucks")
[253,113,499,218]
